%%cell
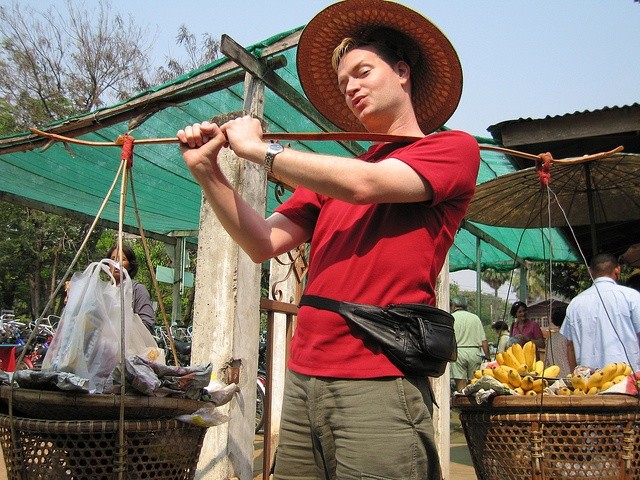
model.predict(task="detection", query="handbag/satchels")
[296,296,458,377]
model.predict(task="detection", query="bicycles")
[0,314,267,434]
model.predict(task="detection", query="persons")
[559,254,639,373]
[511,302,546,362]
[491,321,510,355]
[545,305,576,378]
[450,295,490,393]
[106,243,157,335]
[176,20,481,480]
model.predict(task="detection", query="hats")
[296,0,463,140]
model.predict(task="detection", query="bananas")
[557,361,633,396]
[471,340,560,397]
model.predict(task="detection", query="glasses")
[511,302,526,312]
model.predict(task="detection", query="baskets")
[0,414,208,480]
[460,412,640,480]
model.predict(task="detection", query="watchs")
[263,143,284,174]
